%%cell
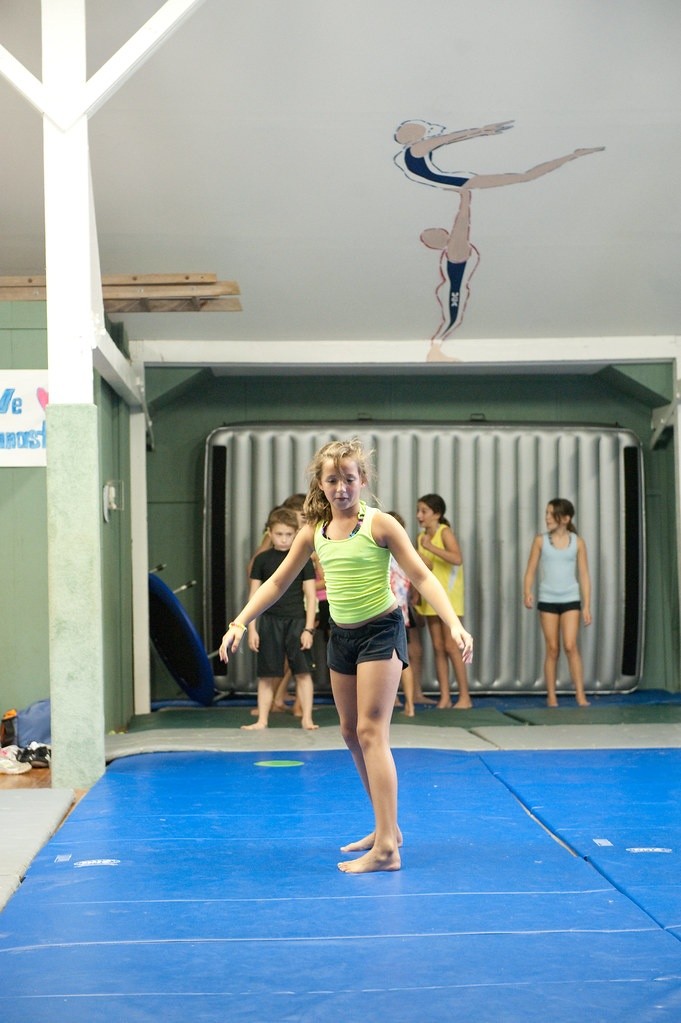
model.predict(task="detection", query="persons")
[241,493,331,729]
[386,494,473,716]
[219,441,473,873]
[523,498,592,708]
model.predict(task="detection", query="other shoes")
[0,740,51,774]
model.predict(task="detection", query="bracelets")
[229,622,247,633]
[304,628,316,634]
